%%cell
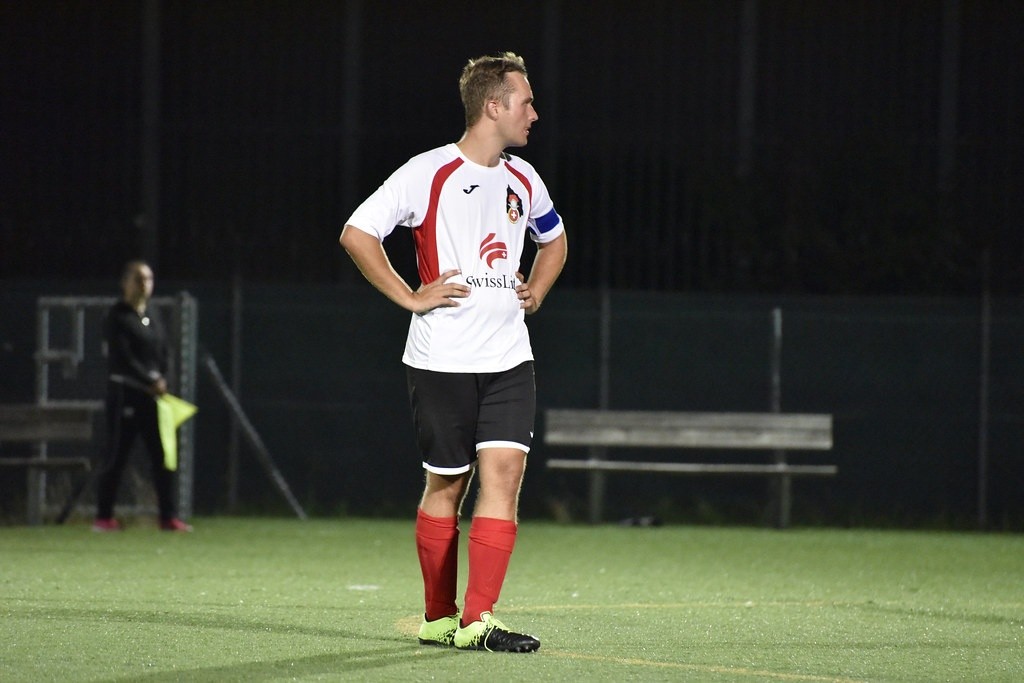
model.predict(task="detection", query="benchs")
[0,399,107,526]
[539,408,838,527]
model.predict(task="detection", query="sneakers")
[418,608,463,645]
[453,610,541,653]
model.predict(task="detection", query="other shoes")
[90,515,120,534]
[162,517,195,532]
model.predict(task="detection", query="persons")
[90,262,194,531]
[339,52,567,652]
